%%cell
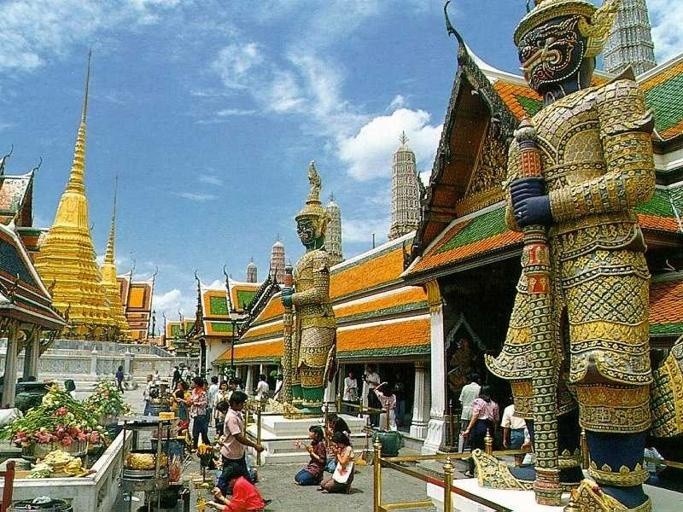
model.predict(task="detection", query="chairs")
[464,469,475,478]
[262,498,273,507]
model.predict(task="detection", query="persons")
[114,365,125,394]
[278,158,337,417]
[371,381,398,431]
[457,371,483,454]
[480,2,657,512]
[500,394,530,450]
[292,413,355,494]
[361,366,381,427]
[460,384,500,477]
[342,368,358,413]
[144,364,271,510]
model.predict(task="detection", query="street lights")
[189,401,208,419]
[331,458,356,485]
[348,386,360,402]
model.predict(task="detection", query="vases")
[5,376,104,449]
[78,375,132,419]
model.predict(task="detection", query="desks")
[226,305,239,376]
